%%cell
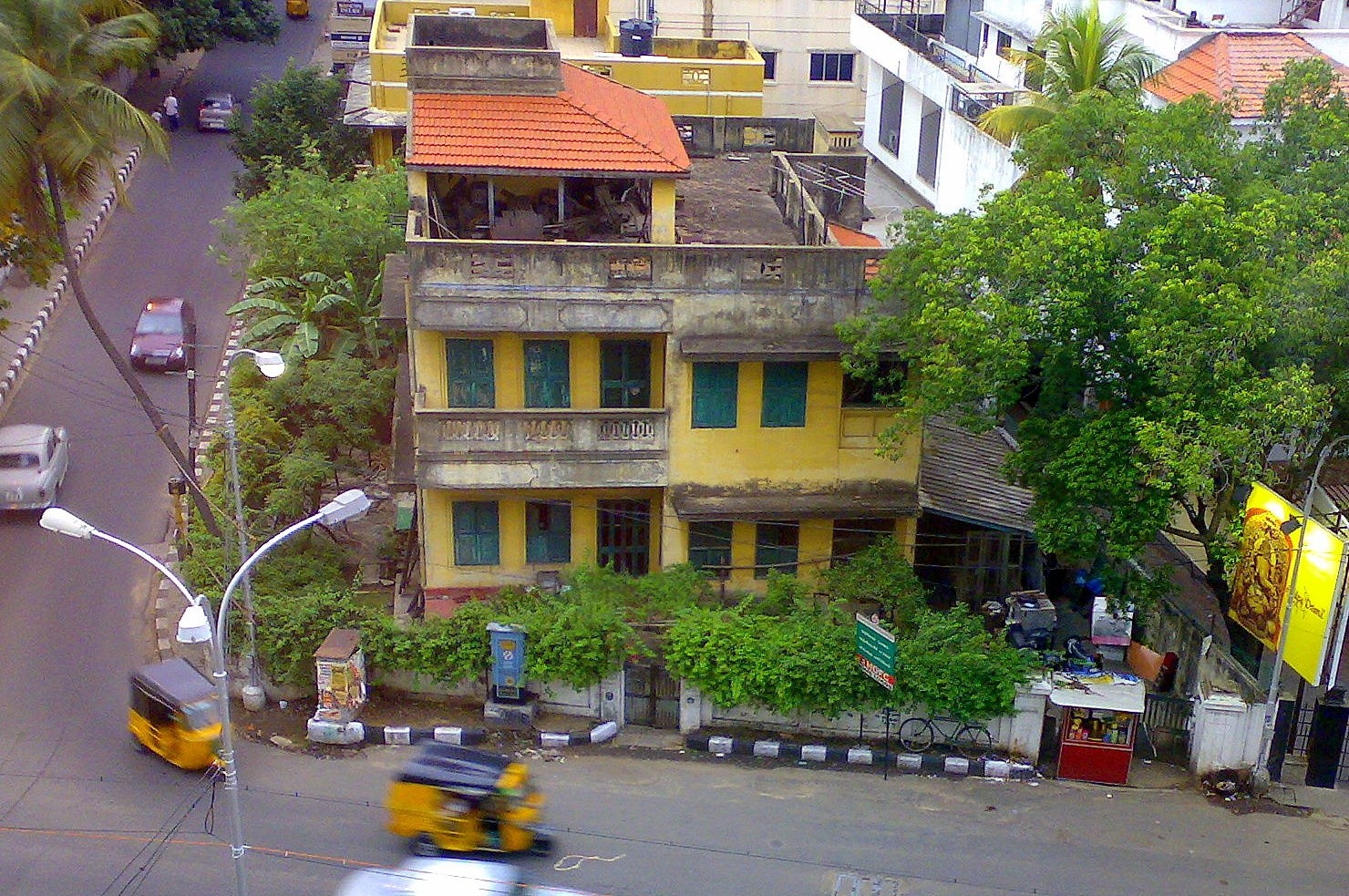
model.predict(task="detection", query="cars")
[127,295,197,373]
[198,90,242,134]
[0,423,68,517]
[332,856,594,896]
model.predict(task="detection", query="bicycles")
[899,707,992,761]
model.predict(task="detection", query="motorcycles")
[286,0,309,20]
[127,656,227,783]
[382,738,556,858]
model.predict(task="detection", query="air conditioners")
[964,98,992,123]
[449,6,475,16]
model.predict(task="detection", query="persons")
[151,108,162,124]
[163,89,179,132]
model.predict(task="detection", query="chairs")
[1009,623,1038,649]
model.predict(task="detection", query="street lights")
[39,489,375,895]
[223,348,285,714]
